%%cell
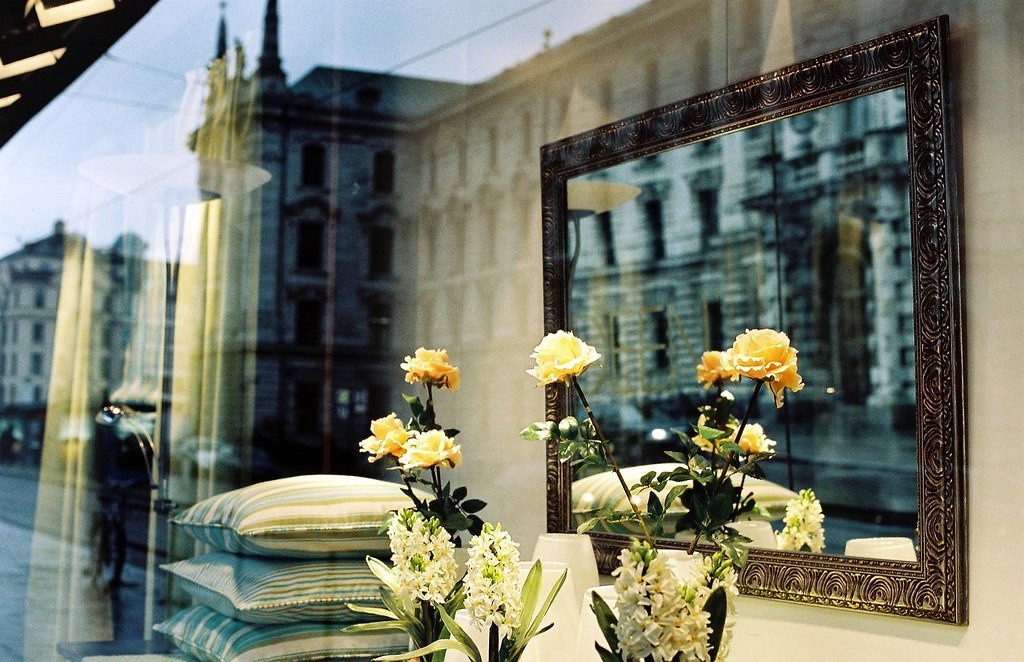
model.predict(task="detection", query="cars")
[581,398,697,466]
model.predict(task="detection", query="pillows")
[571,463,799,539]
[158,550,388,626]
[167,473,437,561]
[153,604,411,662]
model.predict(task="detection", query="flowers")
[341,347,569,662]
[518,329,825,662]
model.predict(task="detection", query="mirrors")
[538,12,968,624]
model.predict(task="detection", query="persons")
[0,423,20,468]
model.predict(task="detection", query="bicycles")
[85,484,158,582]
